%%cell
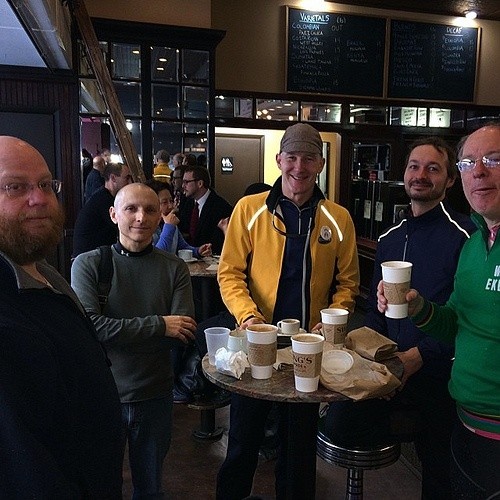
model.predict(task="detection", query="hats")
[280,123,324,154]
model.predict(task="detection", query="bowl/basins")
[206,268,217,274]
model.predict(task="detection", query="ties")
[189,201,199,239]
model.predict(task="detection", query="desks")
[202,335,404,500]
[183,253,221,325]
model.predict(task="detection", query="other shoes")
[172,386,197,405]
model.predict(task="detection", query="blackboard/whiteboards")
[390,16,482,106]
[284,3,388,104]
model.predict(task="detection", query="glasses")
[183,178,198,185]
[457,155,500,173]
[1,179,64,198]
[272,197,314,239]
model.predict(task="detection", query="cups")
[320,308,350,347]
[178,249,192,260]
[277,319,300,335]
[245,324,278,379]
[290,334,325,392]
[228,331,248,355]
[381,261,413,319]
[204,327,231,366]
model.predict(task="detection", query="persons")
[327,138,477,500]
[0,135,123,500]
[151,151,273,403]
[377,124,500,500]
[217,123,360,500]
[72,151,132,257]
[71,183,197,500]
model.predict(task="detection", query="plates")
[184,258,198,262]
[277,329,307,336]
